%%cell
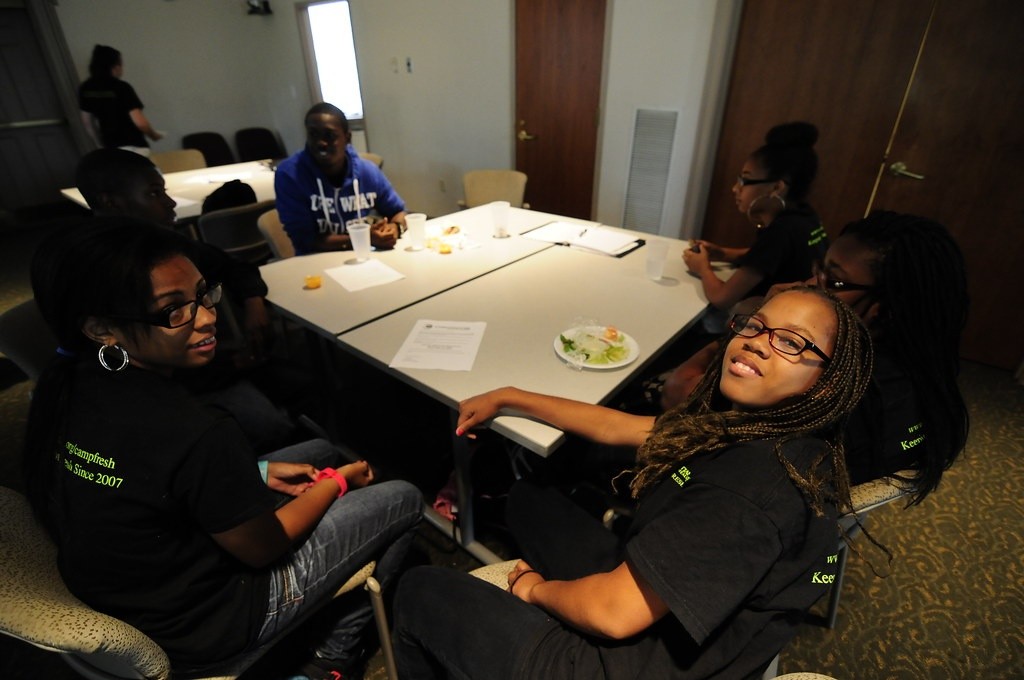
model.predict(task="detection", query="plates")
[554,326,639,369]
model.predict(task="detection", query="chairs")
[0,299,59,384]
[180,131,234,167]
[185,198,279,355]
[827,467,921,627]
[233,127,286,163]
[457,170,529,210]
[0,484,399,680]
[153,149,207,174]
[259,206,298,261]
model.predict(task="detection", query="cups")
[347,224,371,261]
[645,239,669,280]
[491,200,510,236]
[404,213,427,250]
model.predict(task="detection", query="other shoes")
[431,476,474,527]
[283,641,363,680]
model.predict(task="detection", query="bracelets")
[394,222,405,238]
[313,467,348,498]
[510,569,537,593]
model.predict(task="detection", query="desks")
[58,159,278,341]
[337,224,740,566]
[258,199,603,486]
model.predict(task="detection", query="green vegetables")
[560,334,628,364]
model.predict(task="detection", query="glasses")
[731,313,831,366]
[812,262,881,293]
[129,274,226,330]
[738,174,779,187]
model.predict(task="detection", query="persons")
[682,122,832,309]
[78,44,164,157]
[659,209,971,511]
[21,212,426,680]
[393,286,893,679]
[273,102,407,253]
[67,147,275,336]
